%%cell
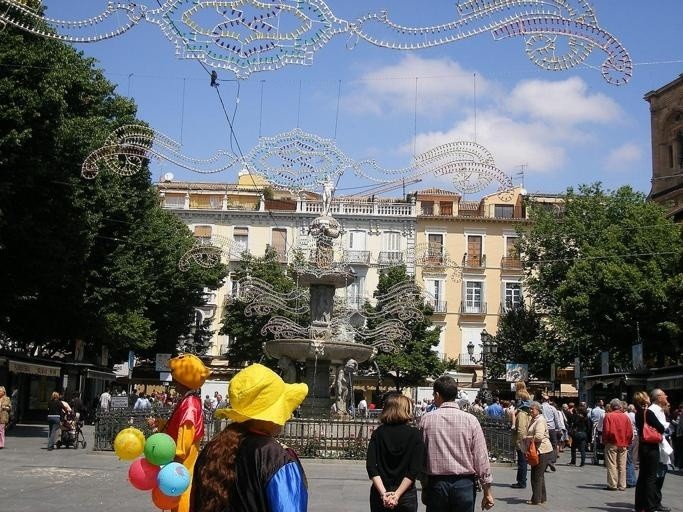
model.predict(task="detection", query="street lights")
[468,329,498,405]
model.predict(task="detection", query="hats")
[215,363,309,426]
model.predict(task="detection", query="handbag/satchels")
[642,422,663,443]
[525,438,539,466]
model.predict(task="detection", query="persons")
[420,375,495,512]
[147,354,212,512]
[358,396,366,417]
[316,175,337,217]
[462,381,683,512]
[412,398,437,426]
[190,363,308,512]
[369,401,376,417]
[0,385,12,448]
[204,390,230,436]
[365,394,425,512]
[46,387,181,451]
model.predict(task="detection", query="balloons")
[152,488,181,510]
[114,428,145,461]
[129,457,162,490]
[144,433,177,466]
[157,462,191,496]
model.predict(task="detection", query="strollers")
[590,424,606,466]
[56,411,89,449]
